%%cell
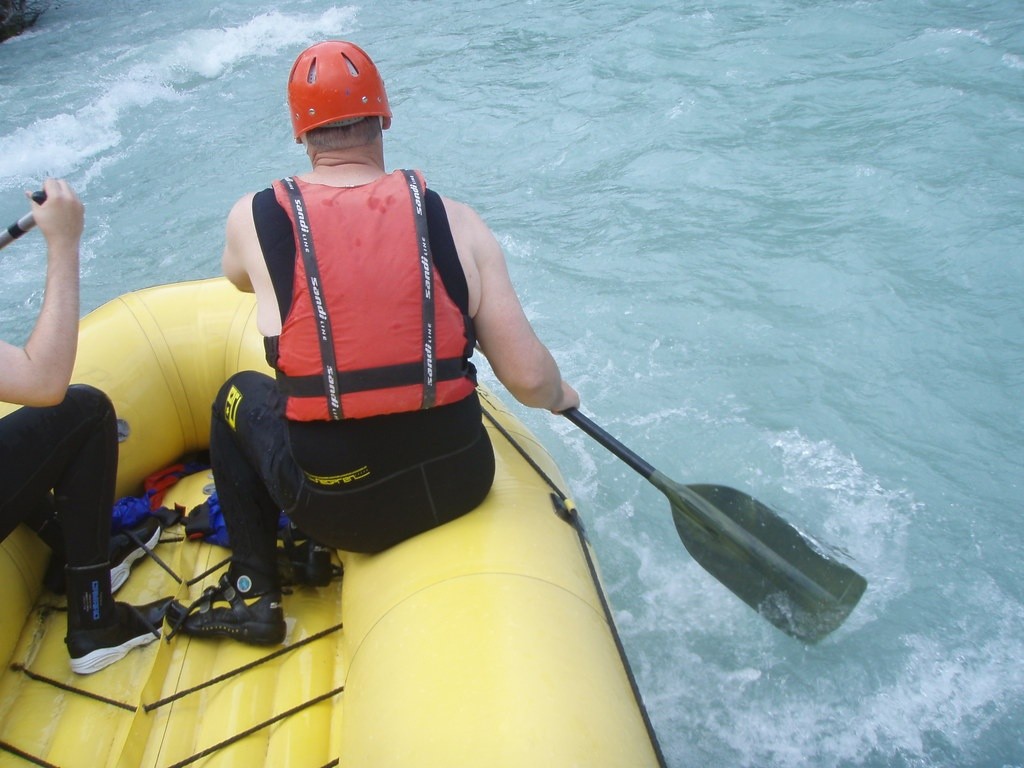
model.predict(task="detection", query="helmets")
[287,39,393,139]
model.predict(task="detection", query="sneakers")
[109,516,163,595]
[165,573,288,647]
[276,519,333,586]
[63,601,175,674]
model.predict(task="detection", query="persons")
[0,177,175,677]
[163,40,579,647]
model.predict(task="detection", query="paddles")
[1,191,46,252]
[474,339,867,647]
